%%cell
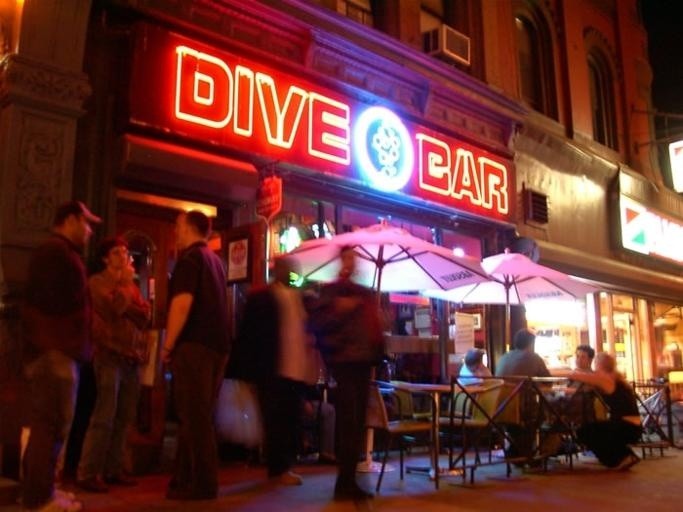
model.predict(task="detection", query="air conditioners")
[424,23,471,69]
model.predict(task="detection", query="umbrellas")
[420,245,611,449]
[264,218,500,462]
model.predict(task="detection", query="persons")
[548,345,596,397]
[459,347,513,456]
[494,330,557,470]
[234,244,389,501]
[546,352,645,473]
[2,194,237,511]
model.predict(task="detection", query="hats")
[56,201,101,225]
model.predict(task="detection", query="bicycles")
[657,389,683,447]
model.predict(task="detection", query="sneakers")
[613,455,640,469]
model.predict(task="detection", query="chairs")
[362,372,540,491]
